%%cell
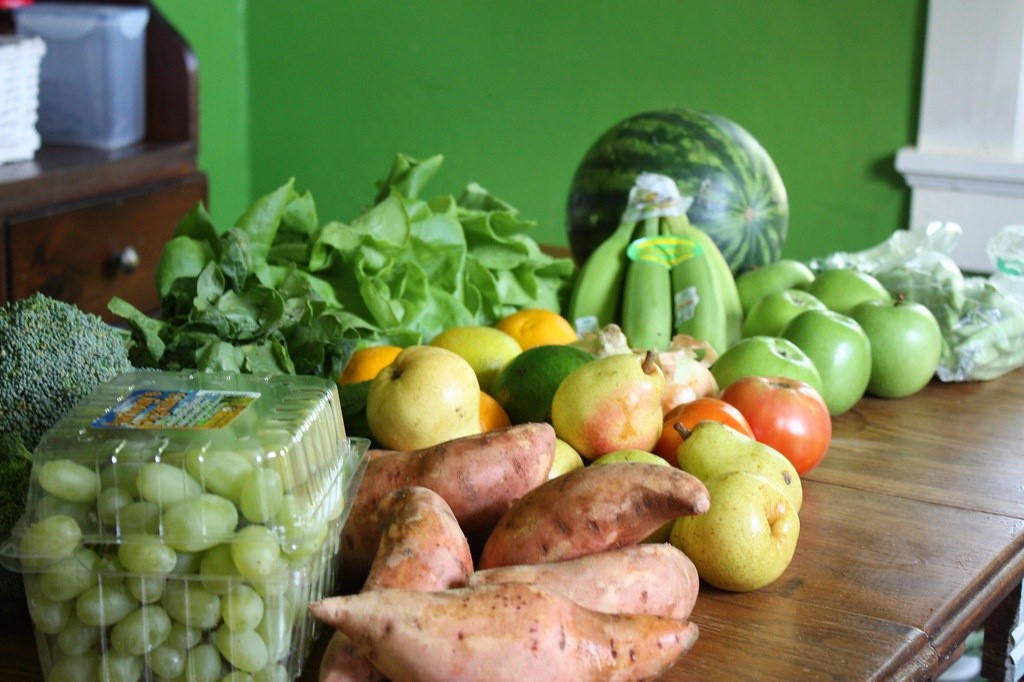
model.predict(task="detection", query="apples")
[710,260,943,417]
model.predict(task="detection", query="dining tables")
[2,241,1024,681]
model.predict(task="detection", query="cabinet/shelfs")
[2,0,213,329]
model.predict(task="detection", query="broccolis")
[0,292,134,534]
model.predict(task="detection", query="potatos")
[307,421,710,681]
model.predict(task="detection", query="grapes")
[15,372,342,681]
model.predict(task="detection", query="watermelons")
[565,107,788,270]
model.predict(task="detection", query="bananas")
[565,173,743,357]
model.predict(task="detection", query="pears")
[366,345,802,593]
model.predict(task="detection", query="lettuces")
[109,154,575,371]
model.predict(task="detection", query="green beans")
[837,249,1024,382]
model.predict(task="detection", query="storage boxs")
[1,354,373,682]
[12,5,159,155]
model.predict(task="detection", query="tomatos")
[658,376,833,476]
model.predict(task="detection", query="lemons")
[341,308,597,433]
